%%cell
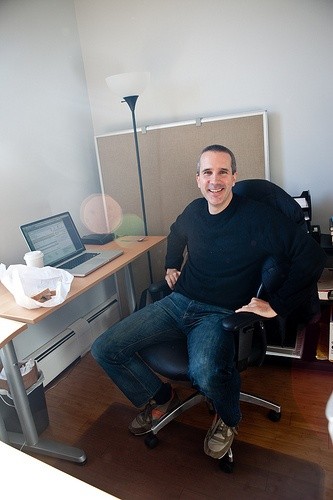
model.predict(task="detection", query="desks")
[0,234,167,500]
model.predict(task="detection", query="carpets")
[54,402,324,500]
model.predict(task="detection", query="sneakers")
[203,418,239,459]
[128,391,182,435]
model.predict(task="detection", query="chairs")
[133,179,306,474]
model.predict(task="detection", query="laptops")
[19,212,124,277]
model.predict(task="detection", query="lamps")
[106,72,154,284]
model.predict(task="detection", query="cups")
[24,251,44,268]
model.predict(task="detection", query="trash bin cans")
[0,370,50,438]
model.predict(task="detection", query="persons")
[90,145,327,459]
